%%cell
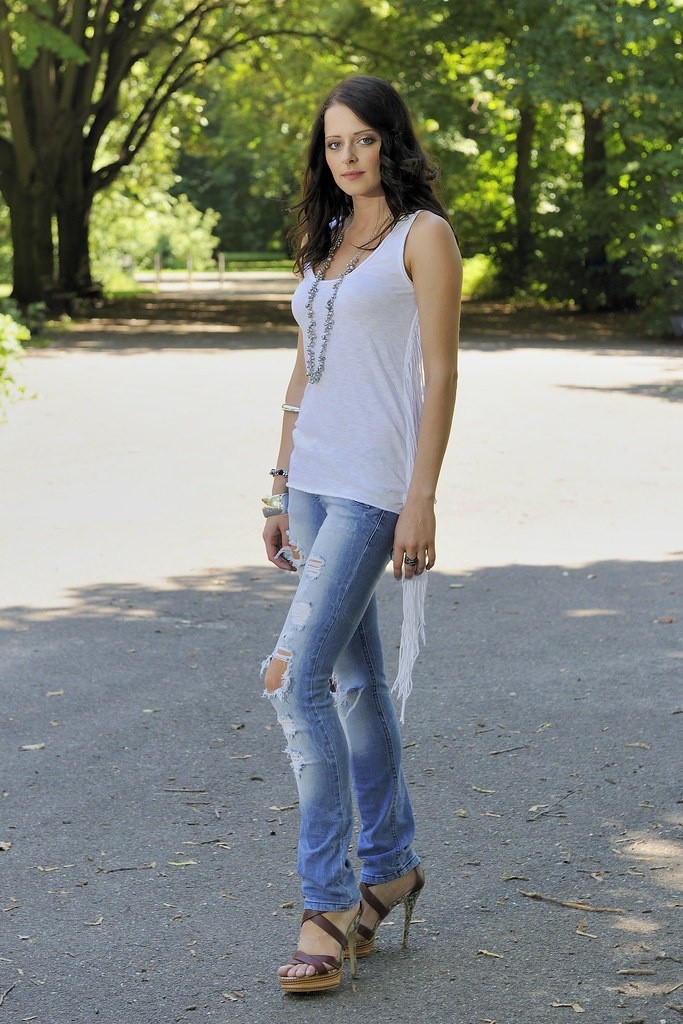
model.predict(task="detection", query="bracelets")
[262,494,288,518]
[268,468,289,477]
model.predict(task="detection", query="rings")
[405,555,417,565]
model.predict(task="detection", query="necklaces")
[305,210,391,384]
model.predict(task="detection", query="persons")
[260,78,464,993]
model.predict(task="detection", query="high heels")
[345,862,425,960]
[281,893,364,992]
